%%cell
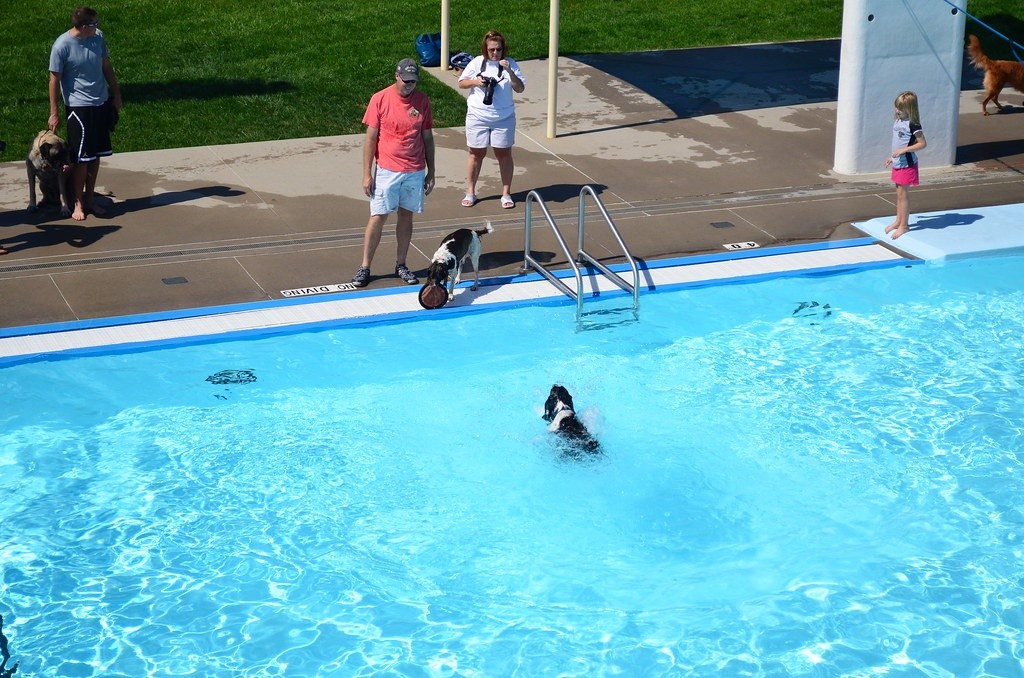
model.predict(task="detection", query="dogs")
[541,384,600,453]
[27,130,88,217]
[968,34,1024,116]
[426,221,494,301]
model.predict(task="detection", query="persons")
[458,30,526,208]
[47,7,122,220]
[884,91,927,239]
[351,59,436,287]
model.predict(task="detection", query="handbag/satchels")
[416,32,440,66]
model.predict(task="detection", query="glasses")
[402,80,416,83]
[87,20,100,28]
[487,47,503,52]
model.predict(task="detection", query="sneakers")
[389,263,420,284]
[350,267,371,286]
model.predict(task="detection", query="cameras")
[482,77,498,105]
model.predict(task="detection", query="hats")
[395,57,419,80]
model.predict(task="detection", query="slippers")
[500,195,514,209]
[461,194,478,206]
